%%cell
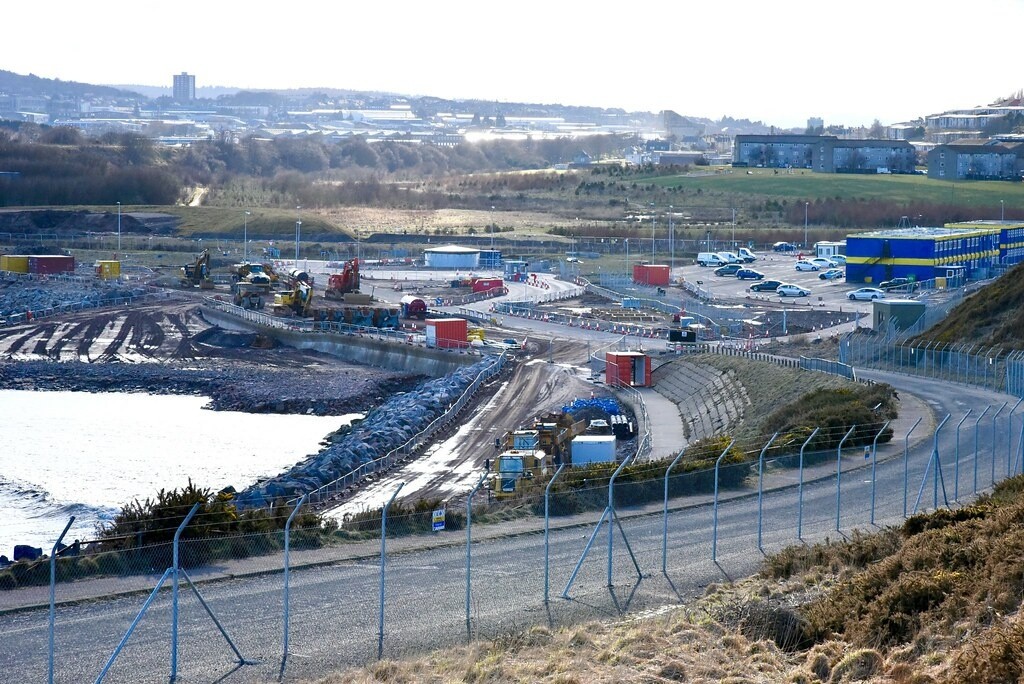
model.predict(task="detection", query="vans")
[697,252,729,267]
[718,251,745,264]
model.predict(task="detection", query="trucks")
[735,247,757,264]
[505,410,586,463]
[230,262,270,285]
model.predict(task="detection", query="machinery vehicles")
[273,272,314,318]
[178,249,215,290]
[324,258,371,305]
[484,430,561,500]
[233,283,265,310]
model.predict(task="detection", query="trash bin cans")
[936,277,947,289]
[908,275,915,282]
[864,277,872,282]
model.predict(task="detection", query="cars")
[845,287,885,301]
[819,269,844,280]
[470,338,522,349]
[750,280,812,297]
[879,278,920,293]
[772,241,796,252]
[795,255,846,271]
[713,264,765,280]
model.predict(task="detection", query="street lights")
[669,205,674,254]
[244,210,250,262]
[296,221,302,268]
[805,202,810,247]
[117,201,121,250]
[490,205,496,249]
